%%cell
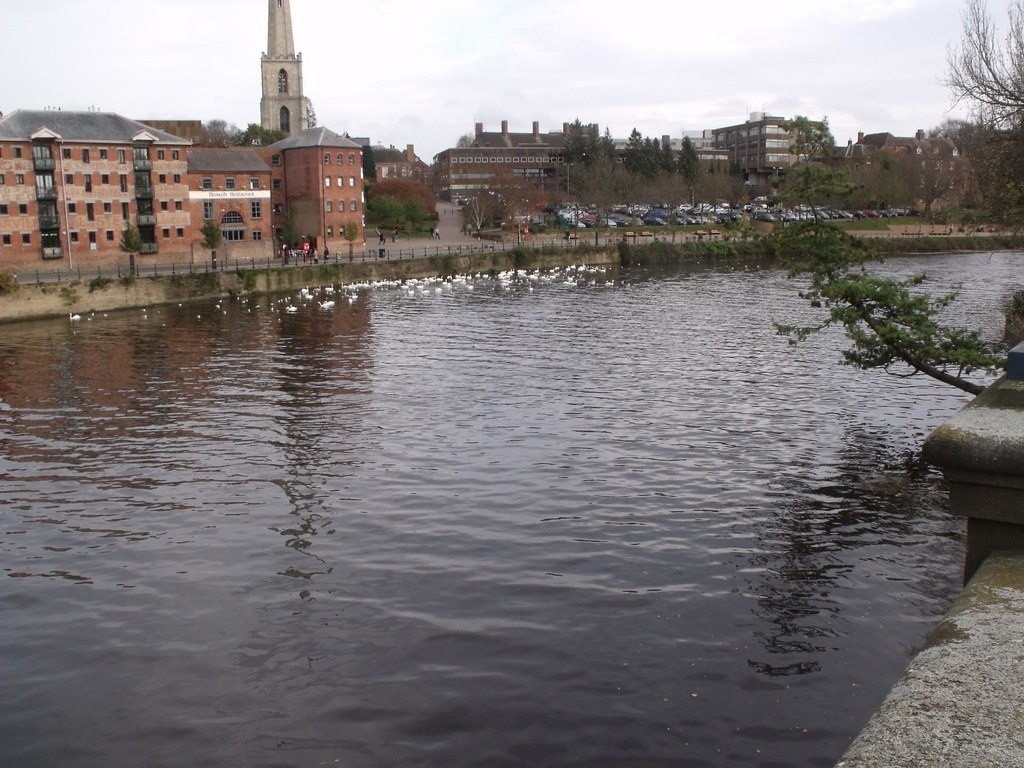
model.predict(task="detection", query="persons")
[565,229,571,243]
[378,233,385,245]
[430,226,434,239]
[312,248,319,264]
[463,223,467,236]
[524,225,528,240]
[434,226,440,239]
[468,223,471,236]
[393,226,400,243]
[324,246,330,264]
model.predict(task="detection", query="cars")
[548,204,921,229]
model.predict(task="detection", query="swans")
[87,261,641,327]
[69,313,80,319]
